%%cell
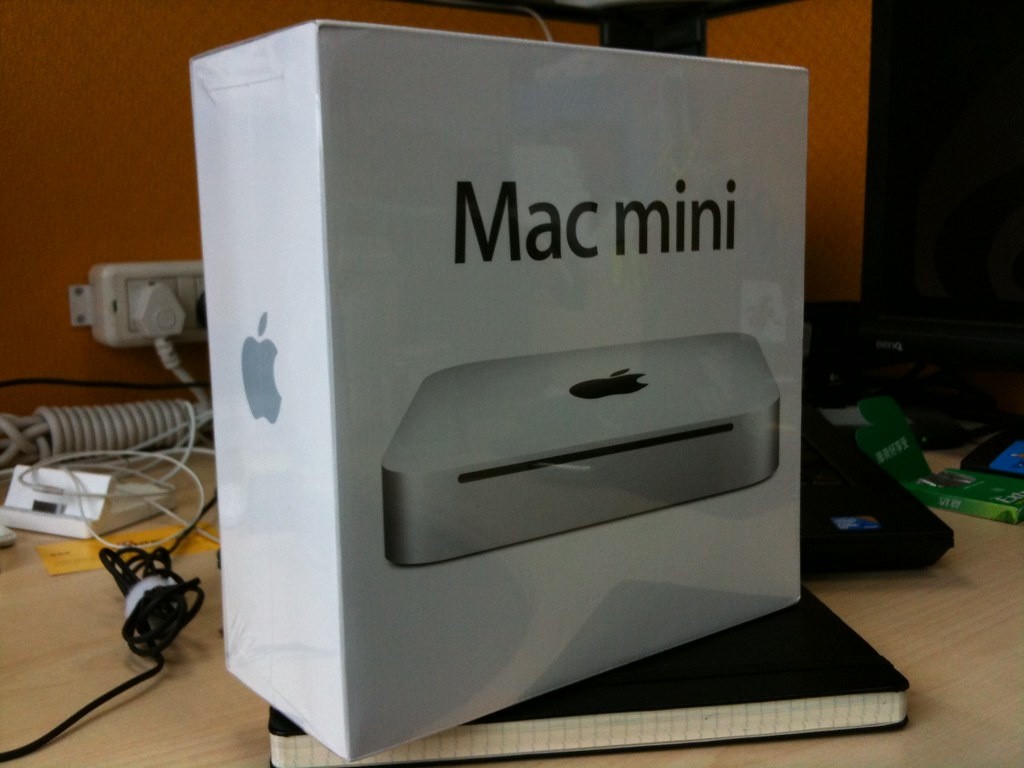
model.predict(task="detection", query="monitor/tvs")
[857,0,1024,374]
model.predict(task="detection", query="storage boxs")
[187,15,810,764]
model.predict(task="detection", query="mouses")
[901,406,946,450]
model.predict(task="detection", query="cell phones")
[960,430,1024,479]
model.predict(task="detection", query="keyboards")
[801,402,954,571]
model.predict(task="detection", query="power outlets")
[67,261,211,351]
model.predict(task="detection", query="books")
[267,577,910,768]
